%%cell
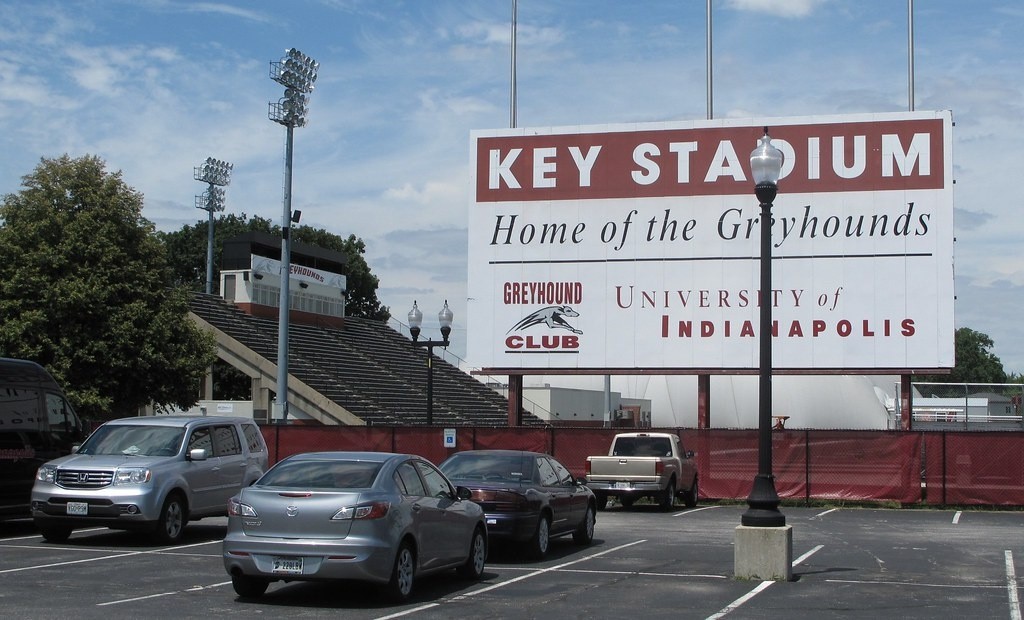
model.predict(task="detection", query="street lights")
[193,156,236,296]
[734,127,798,579]
[409,300,455,425]
[267,48,324,423]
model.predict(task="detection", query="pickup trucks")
[582,431,705,510]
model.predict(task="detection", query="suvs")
[28,415,269,545]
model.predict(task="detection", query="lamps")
[341,291,349,298]
[300,282,309,289]
[254,273,263,280]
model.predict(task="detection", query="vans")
[0,359,88,525]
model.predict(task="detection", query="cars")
[220,452,490,605]
[434,449,600,567]
